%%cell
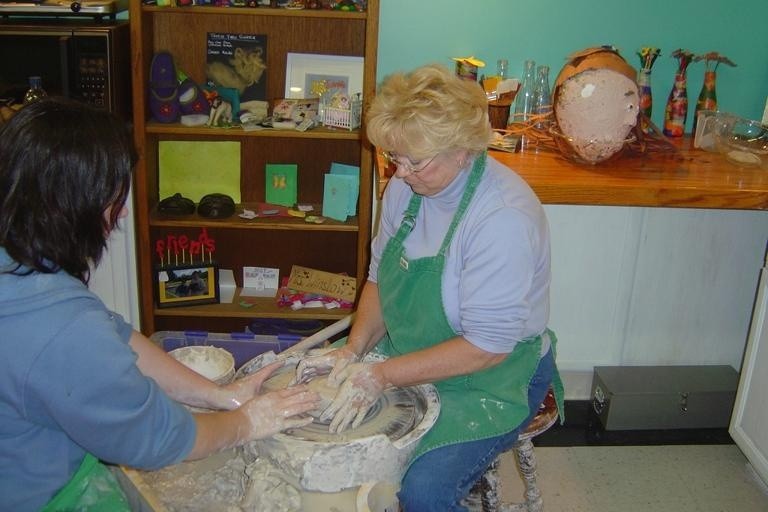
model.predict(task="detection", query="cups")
[694,111,734,154]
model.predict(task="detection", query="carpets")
[459,437,768,510]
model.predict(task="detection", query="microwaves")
[1,15,133,130]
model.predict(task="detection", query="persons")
[289,62,567,511]
[175,275,190,298]
[1,100,322,511]
[189,271,206,296]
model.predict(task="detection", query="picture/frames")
[153,260,223,311]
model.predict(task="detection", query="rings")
[283,408,290,418]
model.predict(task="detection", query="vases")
[663,71,687,137]
[692,70,716,138]
[637,67,653,127]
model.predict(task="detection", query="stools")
[464,387,574,511]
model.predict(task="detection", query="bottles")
[534,64,552,140]
[494,58,511,79]
[515,60,536,133]
[21,72,50,106]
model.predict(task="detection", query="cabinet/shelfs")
[125,0,383,356]
[87,170,140,336]
[478,117,767,407]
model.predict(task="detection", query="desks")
[100,345,441,511]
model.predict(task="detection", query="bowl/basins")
[711,113,768,169]
[227,346,443,490]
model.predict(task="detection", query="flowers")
[669,47,697,72]
[632,44,662,70]
[694,49,738,74]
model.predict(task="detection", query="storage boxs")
[585,360,742,434]
[141,325,331,370]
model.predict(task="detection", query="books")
[261,158,359,223]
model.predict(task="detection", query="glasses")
[376,147,438,174]
